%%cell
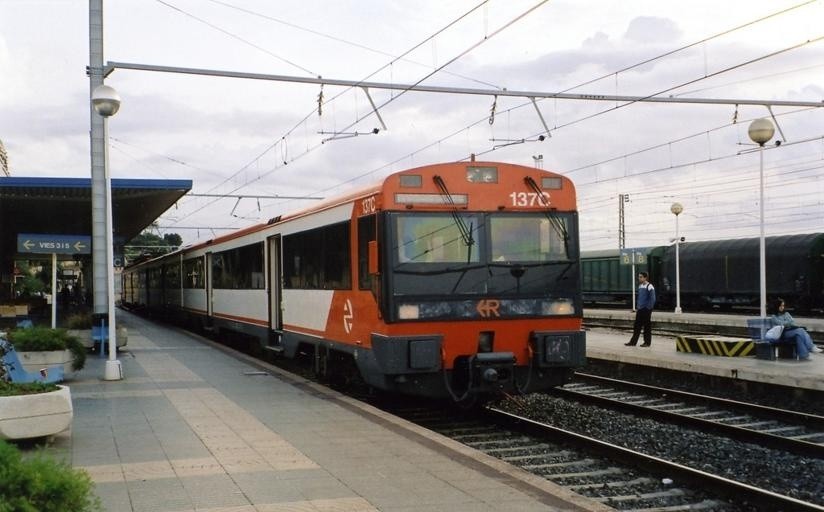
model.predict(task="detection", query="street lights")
[669,202,686,315]
[747,115,776,340]
[93,80,128,384]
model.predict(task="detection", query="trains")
[577,231,824,319]
[116,153,593,419]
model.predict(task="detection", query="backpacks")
[765,324,786,339]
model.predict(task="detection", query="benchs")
[2,345,65,384]
[746,318,799,362]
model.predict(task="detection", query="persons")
[60,283,71,314]
[622,271,656,347]
[769,297,823,361]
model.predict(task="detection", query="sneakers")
[623,342,650,348]
[810,344,822,353]
[804,354,815,361]
